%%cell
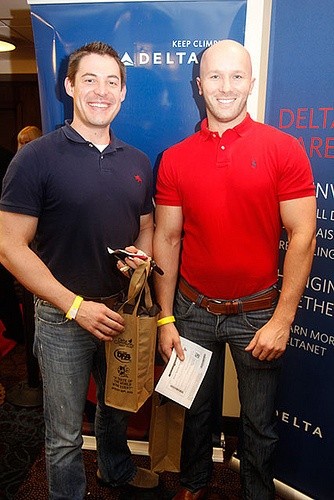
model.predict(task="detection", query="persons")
[0,147,26,344]
[154,39,317,500]
[17,126,43,152]
[0,41,154,500]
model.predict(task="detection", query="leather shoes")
[96,465,167,492]
[172,484,210,500]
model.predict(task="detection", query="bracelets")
[157,315,175,327]
[66,295,83,320]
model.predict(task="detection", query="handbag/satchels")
[105,263,160,414]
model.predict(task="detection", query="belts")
[43,290,128,309]
[179,282,277,315]
